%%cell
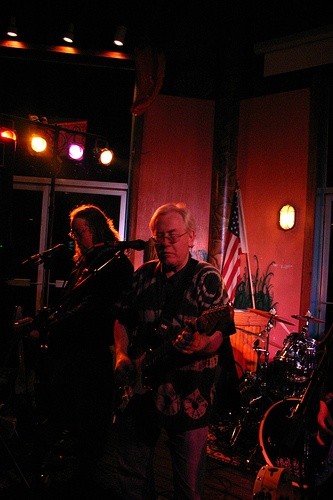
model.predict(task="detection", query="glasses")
[68,226,90,240]
[149,231,190,246]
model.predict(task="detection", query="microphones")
[93,239,146,251]
[21,243,65,265]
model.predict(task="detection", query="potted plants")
[228,255,277,379]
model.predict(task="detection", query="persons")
[113,203,242,500]
[48,204,134,448]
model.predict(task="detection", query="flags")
[221,181,248,304]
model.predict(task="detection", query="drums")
[274,334,324,376]
[252,465,295,500]
[258,398,332,487]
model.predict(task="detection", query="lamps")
[0,113,115,168]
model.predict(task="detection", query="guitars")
[32,303,64,354]
[108,306,230,412]
[7,304,41,409]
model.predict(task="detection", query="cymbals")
[311,300,333,305]
[291,313,326,323]
[246,306,297,327]
[235,326,282,349]
